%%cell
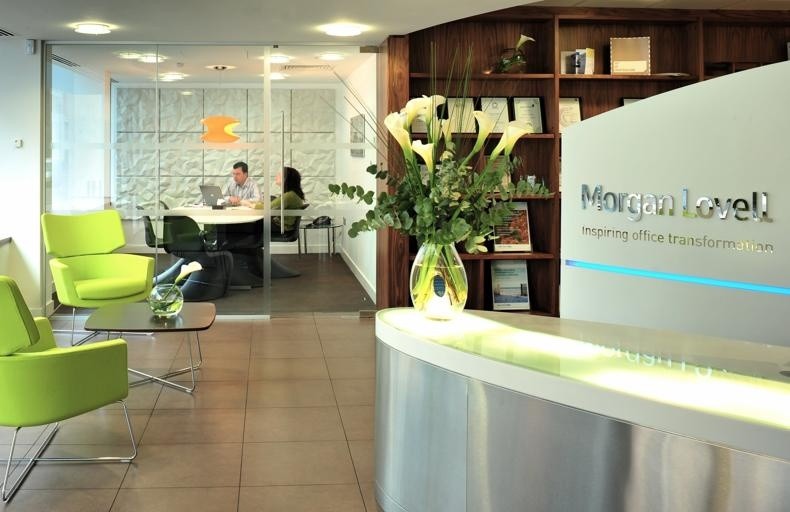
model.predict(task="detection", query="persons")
[240,166,306,236]
[221,162,260,206]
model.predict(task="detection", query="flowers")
[329,79,547,304]
[150,261,203,316]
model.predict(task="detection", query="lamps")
[199,64,240,143]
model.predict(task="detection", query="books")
[608,37,652,77]
[411,97,543,309]
[559,96,582,132]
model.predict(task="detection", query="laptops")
[199,185,232,206]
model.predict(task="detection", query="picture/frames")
[350,115,366,157]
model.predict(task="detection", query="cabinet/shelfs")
[376,8,790,321]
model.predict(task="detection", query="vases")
[408,231,469,316]
[146,284,184,318]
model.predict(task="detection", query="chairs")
[136,195,233,300]
[270,202,309,263]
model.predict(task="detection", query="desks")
[173,205,301,289]
[293,217,346,260]
[84,299,216,394]
